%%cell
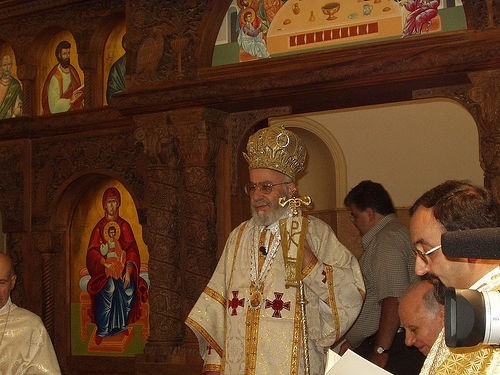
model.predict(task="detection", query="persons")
[399,279,445,355]
[0,253,61,375]
[343,179,421,368]
[185,124,366,375]
[408,180,500,375]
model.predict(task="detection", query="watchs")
[374,346,389,353]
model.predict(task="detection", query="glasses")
[351,212,361,221]
[411,245,442,265]
[245,180,290,195]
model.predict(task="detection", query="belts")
[363,327,404,341]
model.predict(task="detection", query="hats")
[242,123,308,179]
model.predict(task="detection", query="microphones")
[259,246,267,255]
[441,226,500,261]
